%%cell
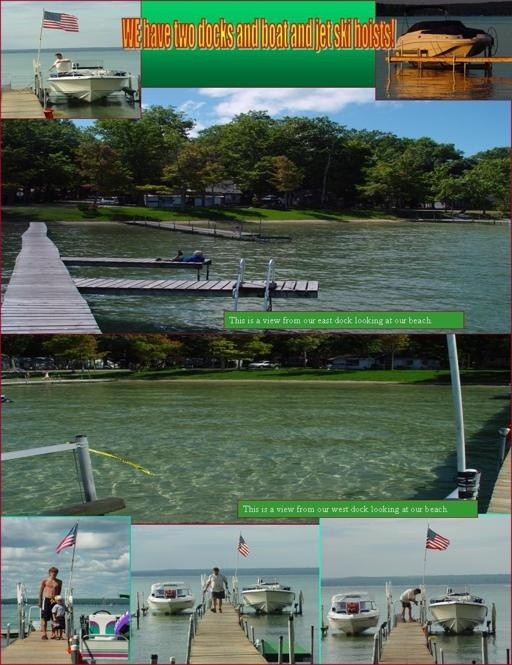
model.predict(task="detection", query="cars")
[248,359,280,369]
[97,195,116,205]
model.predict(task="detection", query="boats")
[429,583,489,634]
[241,577,296,615]
[327,591,380,636]
[393,21,493,66]
[79,610,129,664]
[50,58,133,107]
[147,580,195,614]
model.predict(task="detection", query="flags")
[55,522,79,555]
[238,535,250,557]
[426,527,450,550]
[42,11,78,32]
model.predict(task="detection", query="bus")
[1,354,122,372]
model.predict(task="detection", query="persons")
[204,568,230,614]
[51,596,71,640]
[38,567,62,639]
[400,587,422,620]
[48,54,71,76]
[155,248,205,262]
